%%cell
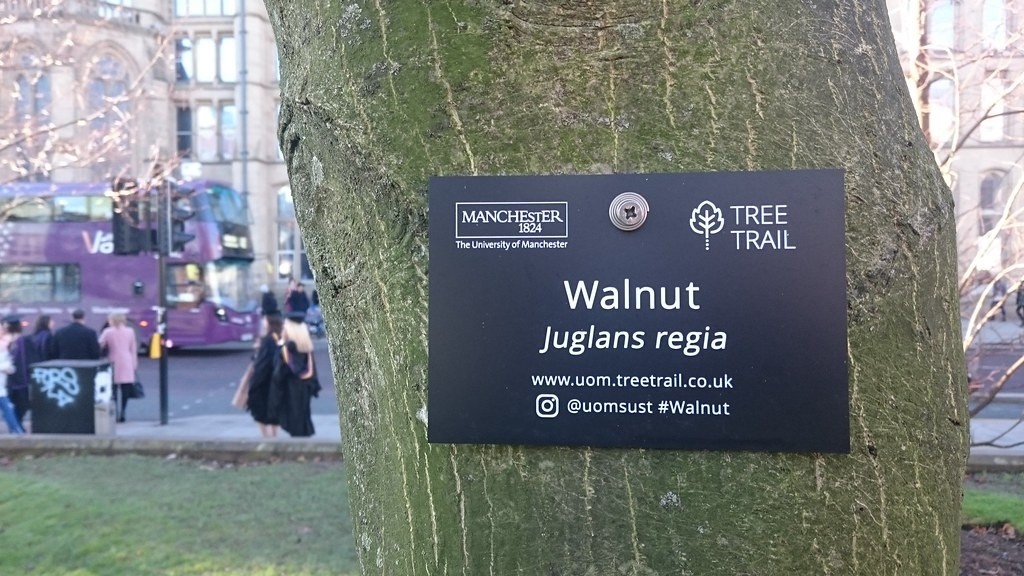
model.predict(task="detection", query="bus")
[0,176,264,356]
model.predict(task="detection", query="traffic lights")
[162,180,194,264]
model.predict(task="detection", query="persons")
[0,308,138,434]
[988,279,1024,328]
[246,277,318,440]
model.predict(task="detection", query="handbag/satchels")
[126,371,144,398]
